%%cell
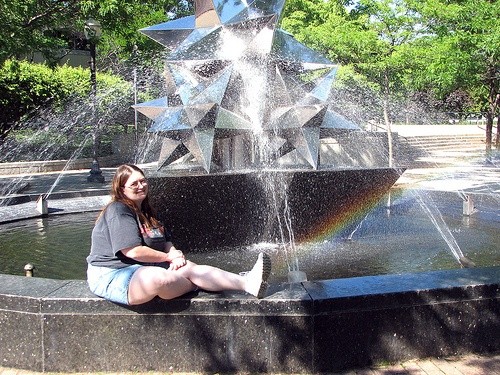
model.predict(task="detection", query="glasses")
[120,179,149,189]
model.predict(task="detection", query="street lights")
[83,17,105,185]
[130,45,143,144]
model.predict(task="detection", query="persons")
[86,164,271,306]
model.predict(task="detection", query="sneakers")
[238,251,272,299]
